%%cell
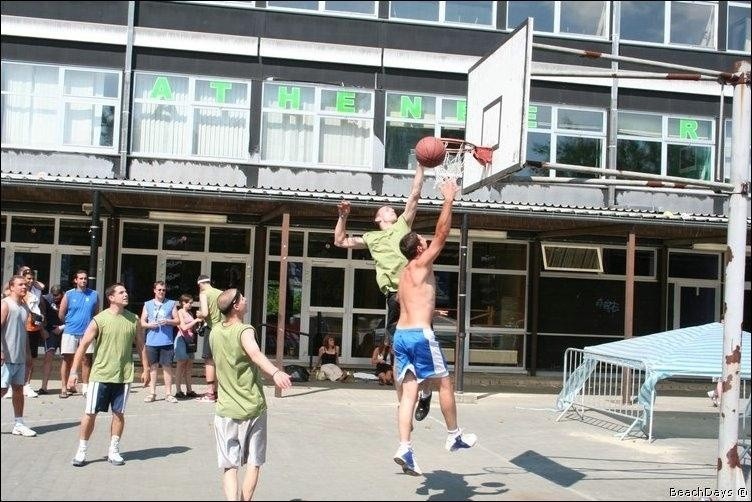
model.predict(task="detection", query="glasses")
[156,289,166,291]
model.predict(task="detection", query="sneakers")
[393,447,421,476]
[11,425,36,438]
[445,433,480,455]
[415,390,434,421]
[71,450,86,466]
[4,389,12,399]
[60,392,218,404]
[108,447,125,464]
[23,385,38,398]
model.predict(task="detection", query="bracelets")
[271,368,279,377]
[69,370,78,375]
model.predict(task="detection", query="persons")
[64,281,152,467]
[1,275,37,436]
[193,275,224,403]
[310,335,350,383]
[208,288,293,501]
[333,159,440,431]
[372,333,393,386]
[0,264,204,405]
[390,177,479,475]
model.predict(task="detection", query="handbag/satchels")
[31,313,42,327]
[183,337,196,354]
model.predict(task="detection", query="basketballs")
[416,136,446,167]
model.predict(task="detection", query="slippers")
[37,388,46,394]
